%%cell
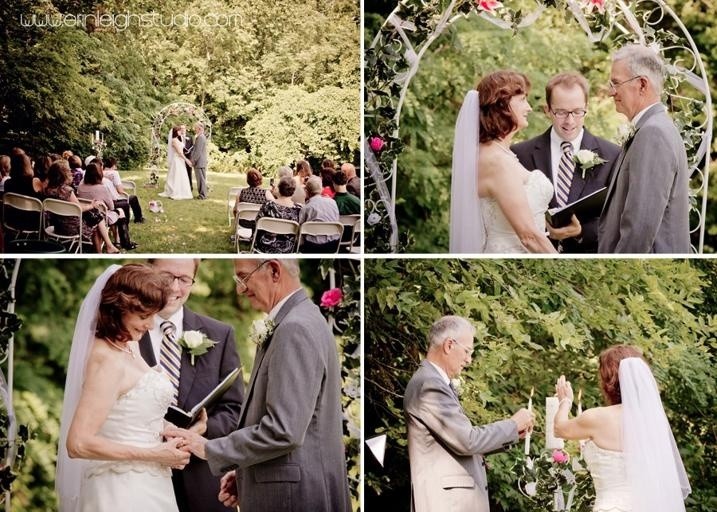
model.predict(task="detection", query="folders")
[167,366,241,430]
[546,183,607,228]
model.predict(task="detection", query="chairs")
[226,184,360,254]
[1,181,136,254]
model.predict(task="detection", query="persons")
[594,44,695,253]
[510,70,622,253]
[449,69,560,255]
[0,147,145,253]
[190,122,206,200]
[158,126,193,200]
[177,124,194,192]
[231,157,360,254]
[553,344,692,512]
[58,257,353,512]
[403,315,535,512]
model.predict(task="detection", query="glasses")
[452,339,476,356]
[549,104,587,120]
[608,75,642,91]
[232,259,279,290]
[155,270,200,290]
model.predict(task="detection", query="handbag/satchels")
[82,208,104,229]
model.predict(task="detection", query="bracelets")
[558,396,573,411]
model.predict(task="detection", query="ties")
[157,319,183,411]
[553,141,584,206]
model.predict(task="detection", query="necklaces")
[491,139,520,162]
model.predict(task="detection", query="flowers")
[519,452,571,502]
[369,136,384,151]
[319,288,343,308]
[247,319,273,349]
[613,124,636,147]
[572,149,605,169]
[475,1,504,16]
[584,0,605,15]
[181,329,215,365]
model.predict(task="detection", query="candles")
[524,386,534,455]
[577,389,583,444]
[545,396,565,449]
